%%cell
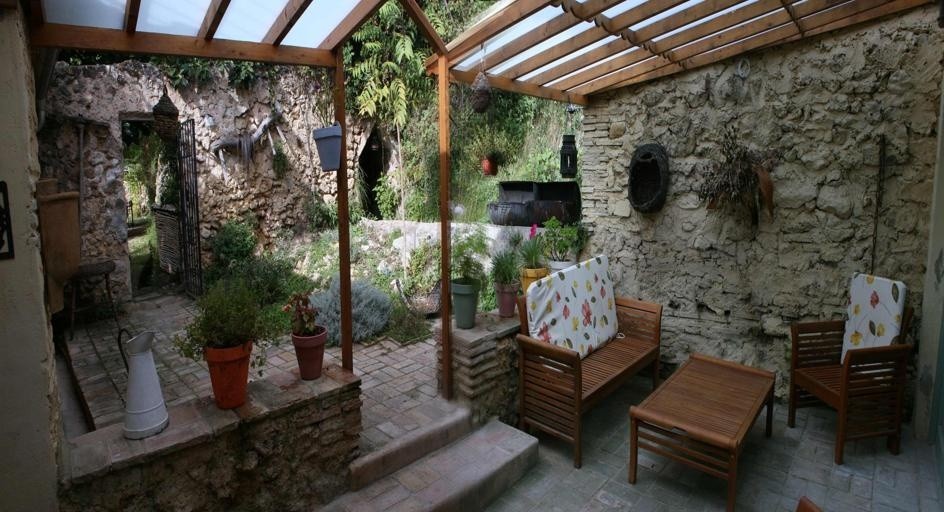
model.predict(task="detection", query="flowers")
[283,286,329,335]
[520,223,546,269]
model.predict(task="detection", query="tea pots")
[116,327,170,440]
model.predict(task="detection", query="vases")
[292,325,328,380]
[521,268,545,296]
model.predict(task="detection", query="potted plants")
[313,75,343,172]
[174,279,273,408]
[457,133,526,176]
[451,228,489,328]
[546,216,581,274]
[463,87,497,116]
[491,249,520,317]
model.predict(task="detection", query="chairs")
[516,294,663,468]
[788,307,913,465]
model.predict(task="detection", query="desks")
[57,260,122,341]
